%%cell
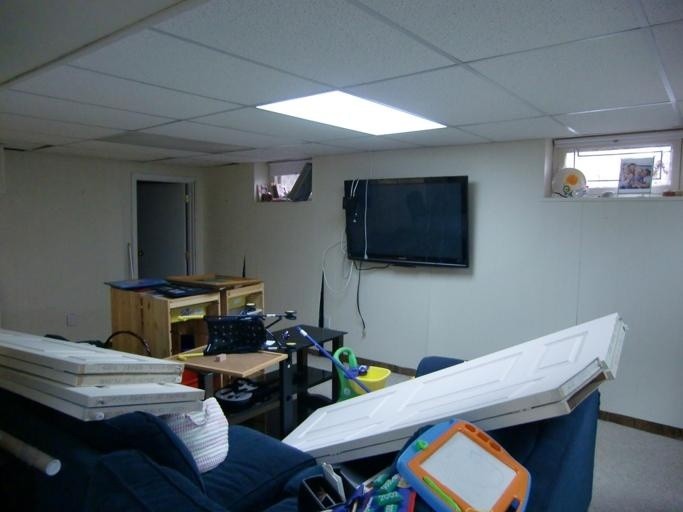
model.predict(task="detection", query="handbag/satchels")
[159,397,230,475]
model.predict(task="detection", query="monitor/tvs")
[344,175,470,268]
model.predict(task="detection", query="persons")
[629,169,642,189]
[625,163,638,187]
[636,168,651,188]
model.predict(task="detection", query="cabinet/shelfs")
[109,277,348,444]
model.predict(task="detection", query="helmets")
[551,168,586,198]
